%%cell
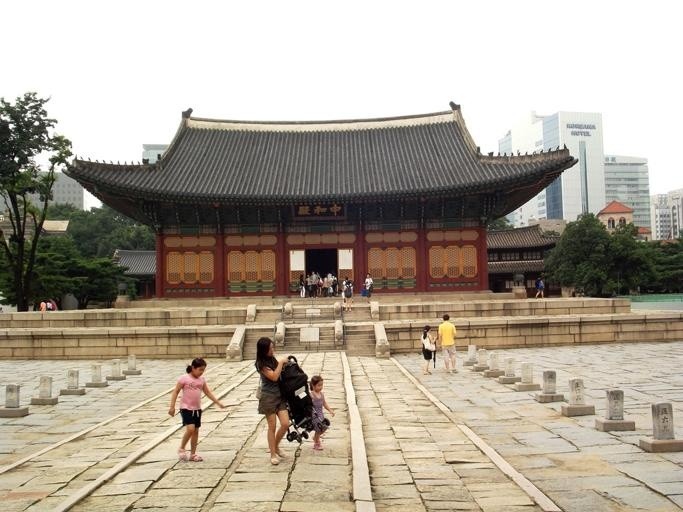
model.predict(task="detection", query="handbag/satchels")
[361,289,368,296]
[256,387,262,399]
[370,286,373,291]
[425,341,436,352]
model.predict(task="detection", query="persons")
[438,315,459,373]
[46,301,52,310]
[309,376,335,450]
[39,300,46,311]
[169,358,226,462]
[300,271,354,312]
[255,338,289,465]
[421,326,439,375]
[363,273,373,304]
[535,275,545,299]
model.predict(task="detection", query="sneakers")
[424,368,458,376]
[270,448,287,465]
[178,449,186,461]
[313,436,324,452]
[189,454,202,461]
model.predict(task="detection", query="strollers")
[276,354,330,445]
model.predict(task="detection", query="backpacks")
[345,286,352,298]
